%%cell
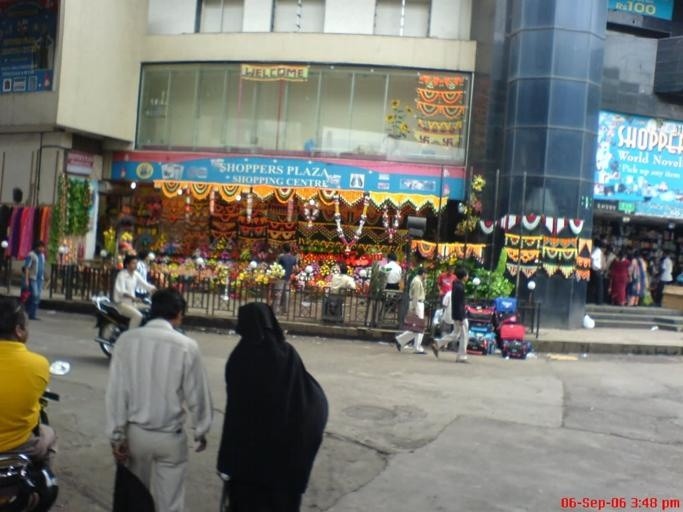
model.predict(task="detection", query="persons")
[32,15,56,71]
[436,266,457,308]
[270,242,300,313]
[211,299,330,509]
[439,289,457,352]
[110,254,159,332]
[101,287,216,511]
[378,252,404,312]
[19,18,30,39]
[0,294,58,477]
[131,247,150,298]
[393,268,428,355]
[430,307,444,341]
[428,267,471,365]
[17,238,46,321]
[589,239,673,308]
[318,265,357,325]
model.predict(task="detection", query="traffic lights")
[406,216,427,237]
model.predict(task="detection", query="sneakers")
[412,349,427,355]
[430,341,440,359]
[393,336,402,352]
[455,358,471,364]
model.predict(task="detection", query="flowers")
[102,226,371,301]
[387,100,413,137]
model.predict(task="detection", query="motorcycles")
[0,359,72,511]
[90,287,186,358]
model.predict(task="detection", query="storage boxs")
[661,285,682,310]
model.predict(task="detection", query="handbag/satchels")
[403,314,426,334]
